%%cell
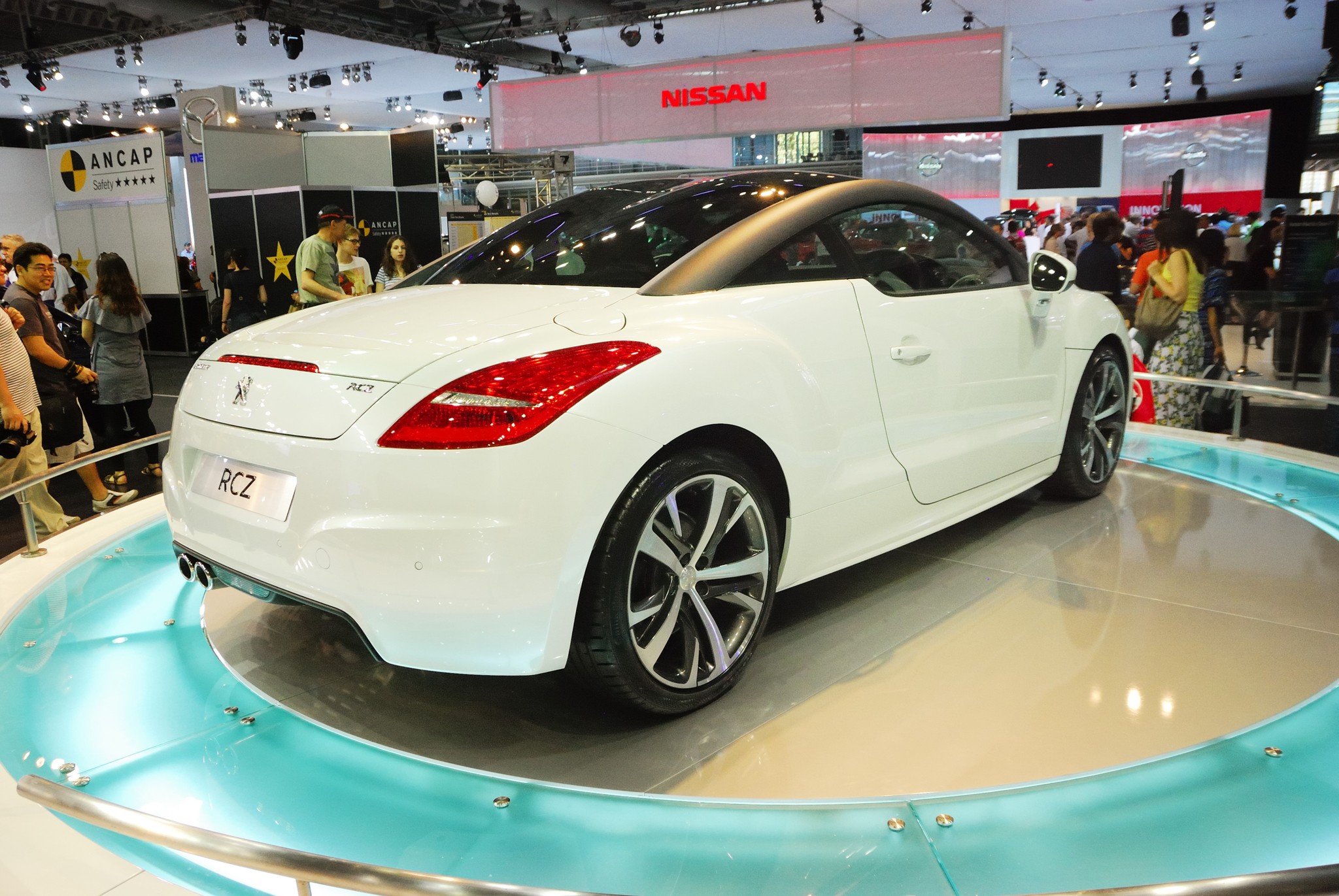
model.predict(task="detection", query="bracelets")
[222,321,227,324]
[295,296,299,301]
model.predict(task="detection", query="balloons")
[475,180,499,207]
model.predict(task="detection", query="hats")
[318,205,355,220]
[184,242,190,246]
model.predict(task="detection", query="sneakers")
[64,516,80,525]
[92,489,139,513]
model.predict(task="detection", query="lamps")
[288,69,331,92]
[21,56,63,91]
[424,0,587,89]
[239,79,272,108]
[20,95,90,132]
[1038,4,1243,110]
[280,24,305,59]
[0,67,11,88]
[133,77,185,115]
[341,62,373,85]
[812,0,974,43]
[114,45,145,69]
[1284,0,1298,19]
[340,90,491,151]
[620,22,642,47]
[268,21,280,47]
[274,106,331,131]
[651,17,665,44]
[234,19,247,46]
[101,102,123,136]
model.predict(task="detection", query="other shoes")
[1234,368,1263,377]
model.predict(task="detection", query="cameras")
[80,381,100,401]
[0,421,37,459]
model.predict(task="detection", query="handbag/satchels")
[1133,250,1189,340]
[1198,360,1249,432]
[37,391,88,457]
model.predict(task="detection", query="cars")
[159,169,1131,720]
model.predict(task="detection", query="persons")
[296,204,355,310]
[222,247,268,335]
[175,242,198,290]
[374,234,423,293]
[291,290,302,310]
[0,233,88,322]
[1,242,139,514]
[75,252,163,485]
[0,249,69,541]
[984,206,1323,369]
[1146,211,1211,431]
[209,245,218,298]
[333,223,374,297]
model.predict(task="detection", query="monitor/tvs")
[1170,168,1184,215]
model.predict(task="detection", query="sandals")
[142,467,162,476]
[104,474,127,485]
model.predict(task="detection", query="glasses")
[27,266,56,272]
[344,238,361,244]
[99,252,118,260]
